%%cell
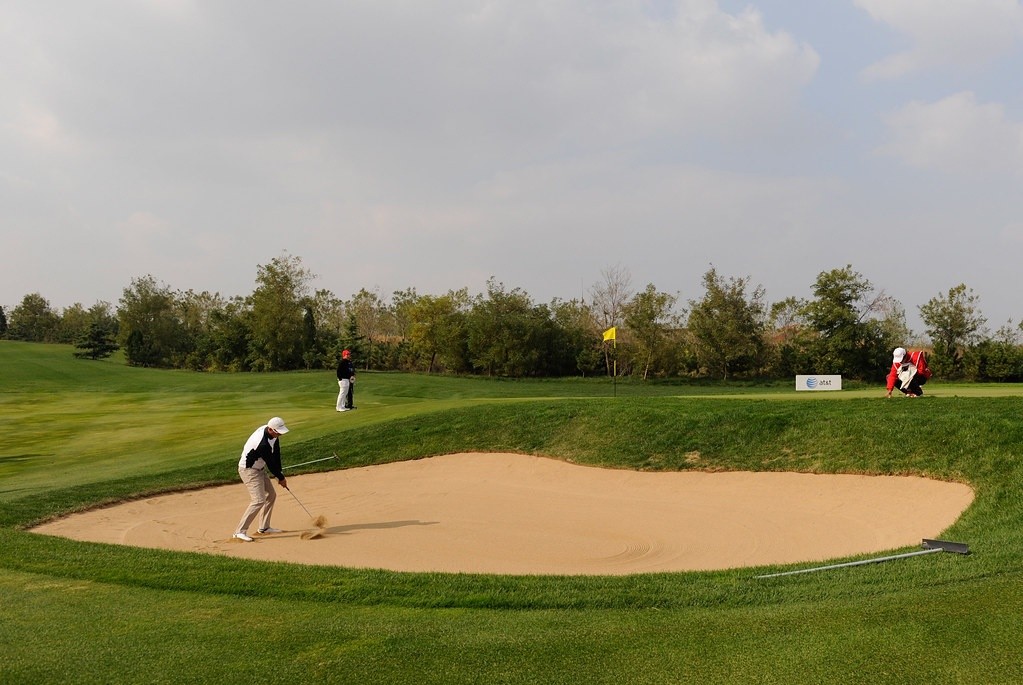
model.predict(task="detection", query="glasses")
[273,430,281,436]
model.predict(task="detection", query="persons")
[335,350,357,412]
[885,348,932,397]
[233,417,290,542]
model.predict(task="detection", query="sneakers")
[257,527,281,533]
[233,533,253,541]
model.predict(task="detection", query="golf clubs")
[273,475,313,520]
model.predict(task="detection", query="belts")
[339,379,342,380]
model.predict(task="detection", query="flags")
[602,327,616,342]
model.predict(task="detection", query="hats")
[342,350,350,356]
[268,417,289,435]
[893,348,906,363]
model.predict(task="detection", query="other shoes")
[910,394,920,397]
[906,391,911,397]
[337,409,345,412]
[344,408,350,410]
